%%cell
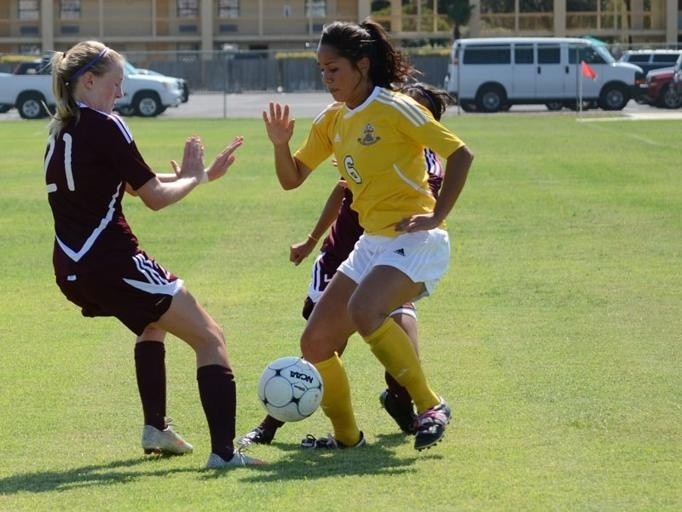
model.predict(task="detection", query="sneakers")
[206,451,272,470]
[414,391,451,451]
[300,430,368,450]
[380,388,419,435]
[237,427,272,446]
[141,423,195,455]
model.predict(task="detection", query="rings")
[407,220,410,224]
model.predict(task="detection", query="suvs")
[617,49,682,110]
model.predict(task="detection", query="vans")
[445,37,643,110]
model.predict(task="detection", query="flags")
[583,62,597,80]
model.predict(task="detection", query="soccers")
[258,356,323,422]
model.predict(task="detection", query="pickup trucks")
[0,58,188,119]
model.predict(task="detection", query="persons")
[237,79,460,447]
[42,39,270,467]
[260,16,475,451]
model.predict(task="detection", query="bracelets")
[307,234,319,242]
